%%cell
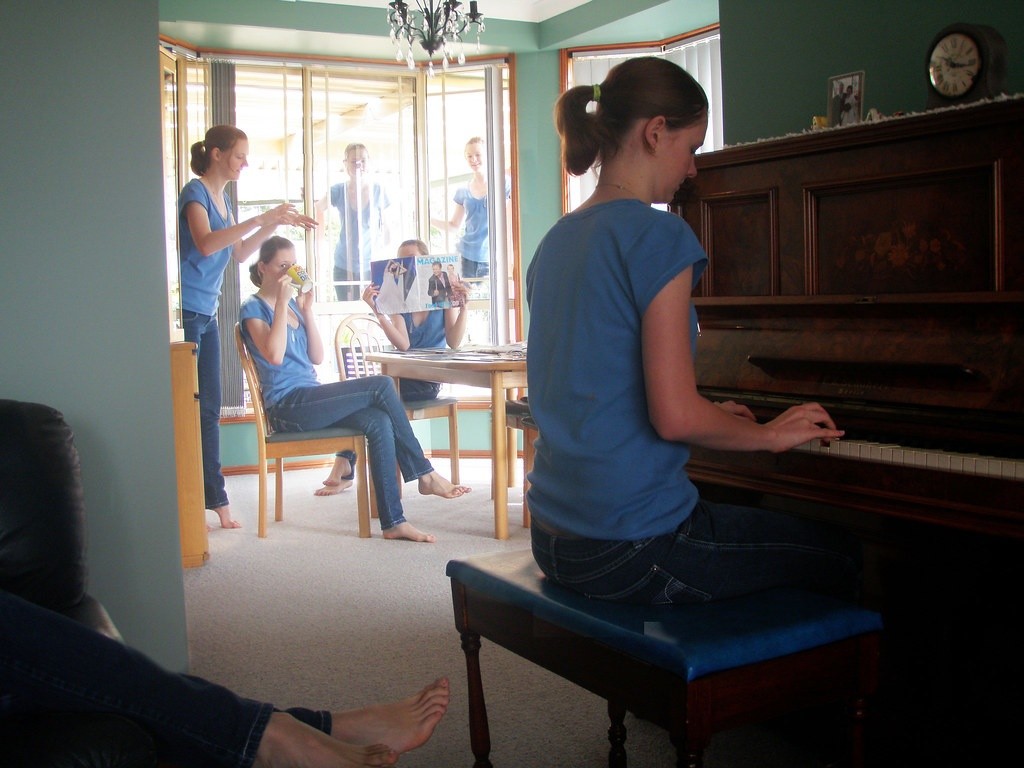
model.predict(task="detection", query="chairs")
[334,314,461,500]
[504,398,547,529]
[234,321,382,539]
[0,400,129,641]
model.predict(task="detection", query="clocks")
[924,24,1008,110]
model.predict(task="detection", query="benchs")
[446,549,897,768]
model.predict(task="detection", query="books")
[370,254,463,315]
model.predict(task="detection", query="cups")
[284,264,314,293]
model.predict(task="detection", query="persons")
[179,125,320,528]
[427,261,461,304]
[238,236,473,544]
[0,586,451,768]
[383,259,408,313]
[525,57,866,606]
[833,81,858,127]
[301,143,392,300]
[312,240,473,495]
[430,136,511,279]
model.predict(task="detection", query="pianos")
[653,105,1024,540]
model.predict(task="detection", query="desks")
[361,348,527,540]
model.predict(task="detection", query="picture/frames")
[826,71,865,127]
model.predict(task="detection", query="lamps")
[386,0,486,77]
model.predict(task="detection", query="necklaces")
[595,184,640,200]
